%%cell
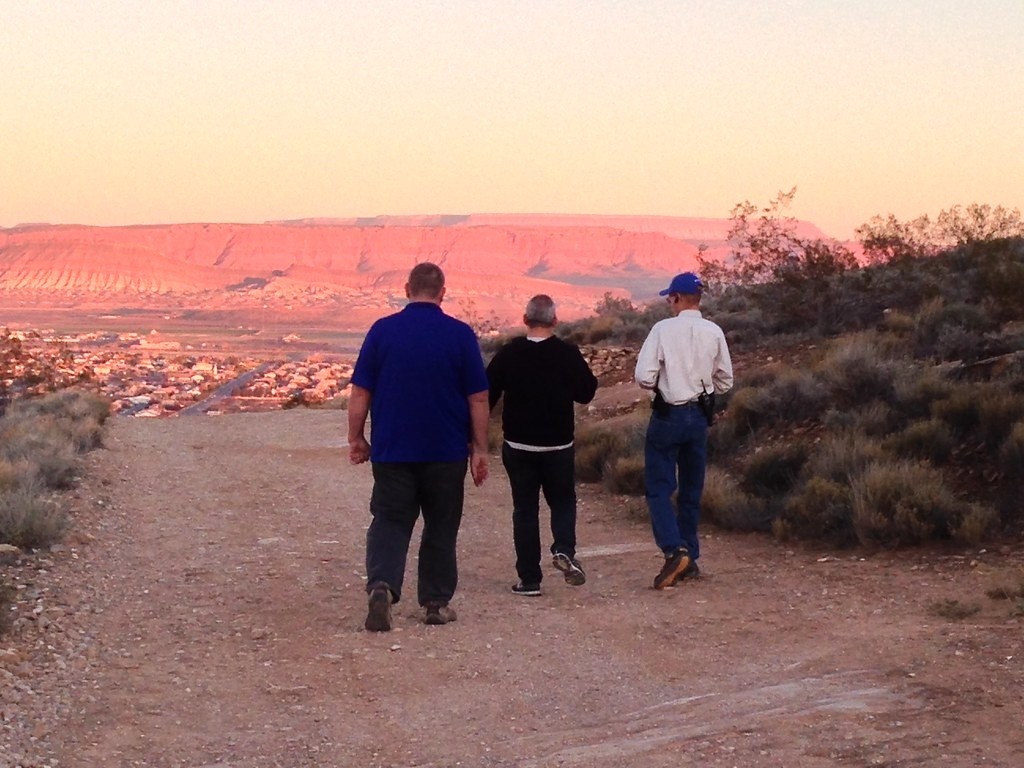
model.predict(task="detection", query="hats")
[659,272,704,295]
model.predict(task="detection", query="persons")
[348,262,490,631]
[486,295,598,594]
[635,272,734,588]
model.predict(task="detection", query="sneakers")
[654,546,690,589]
[552,550,586,586]
[674,562,701,580]
[511,581,540,596]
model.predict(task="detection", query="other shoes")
[424,606,457,624]
[365,587,392,632]
